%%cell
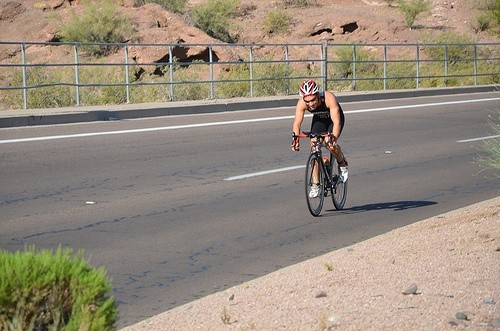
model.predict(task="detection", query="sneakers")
[337,160,349,183]
[308,184,319,198]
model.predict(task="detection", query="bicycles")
[291,131,349,217]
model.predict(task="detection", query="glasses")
[304,96,316,103]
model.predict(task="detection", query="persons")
[291,80,348,198]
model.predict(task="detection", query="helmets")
[299,79,318,97]
